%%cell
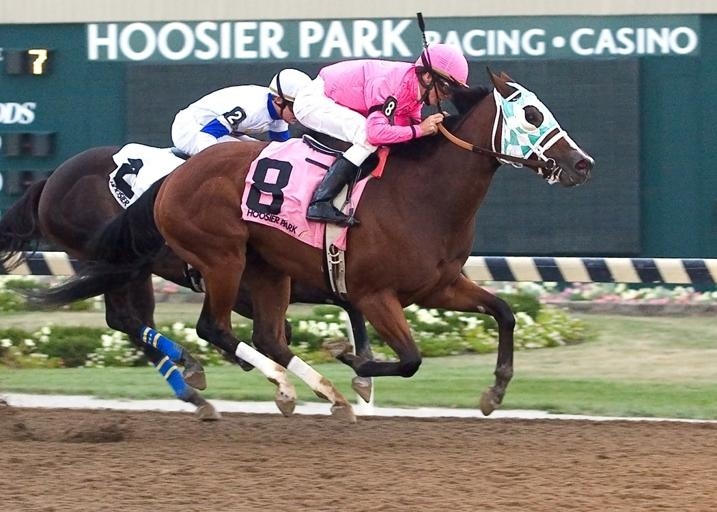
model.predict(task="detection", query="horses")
[7,65,596,425]
[0,145,374,420]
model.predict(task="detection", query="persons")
[293,44,469,225]
[171,68,313,156]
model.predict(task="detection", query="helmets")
[269,68,312,103]
[415,43,470,90]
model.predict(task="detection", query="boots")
[305,155,362,227]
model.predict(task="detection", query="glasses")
[433,76,456,96]
